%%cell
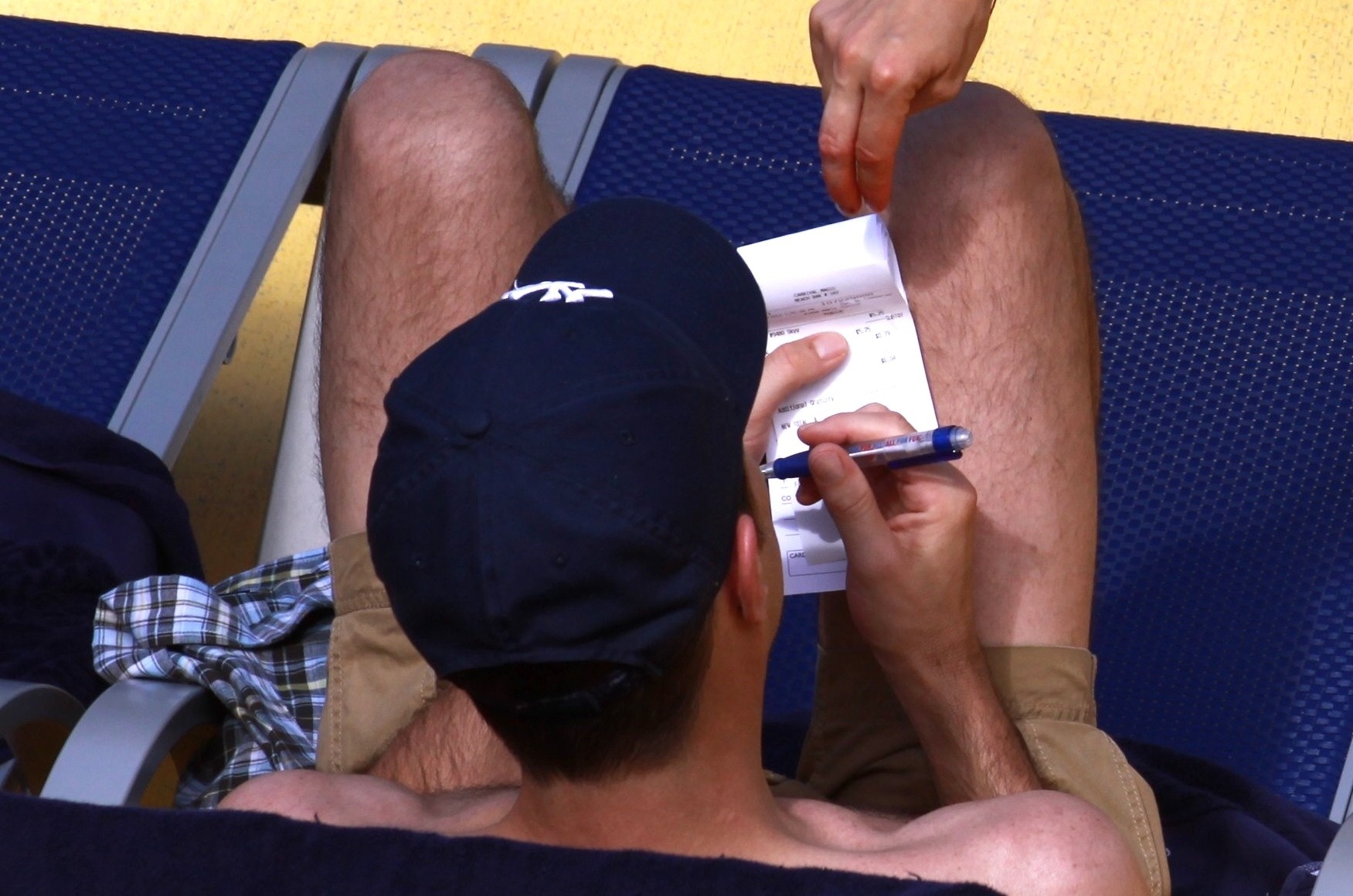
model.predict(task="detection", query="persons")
[806,0,1002,220]
[212,47,1180,894]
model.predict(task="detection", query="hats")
[364,195,769,708]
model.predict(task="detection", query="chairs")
[1,15,374,787]
[0,37,1351,895]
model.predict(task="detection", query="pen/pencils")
[756,425,973,480]
